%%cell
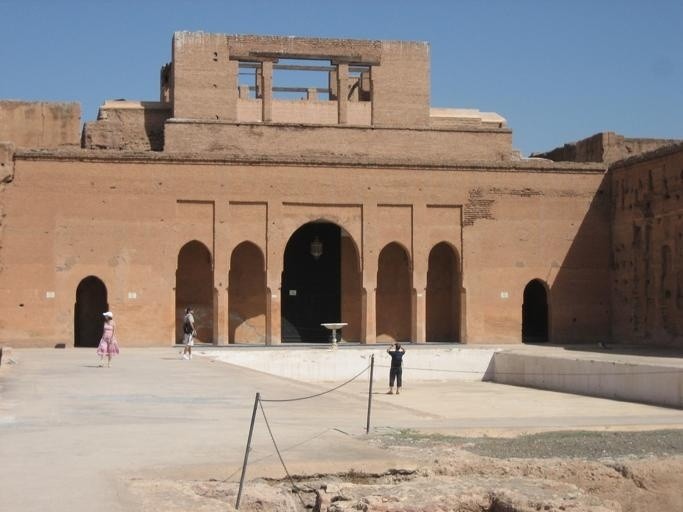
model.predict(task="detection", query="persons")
[387,344,405,394]
[96,311,119,368]
[181,305,197,361]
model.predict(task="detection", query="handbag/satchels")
[183,315,193,334]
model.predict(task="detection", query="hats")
[102,312,112,318]
